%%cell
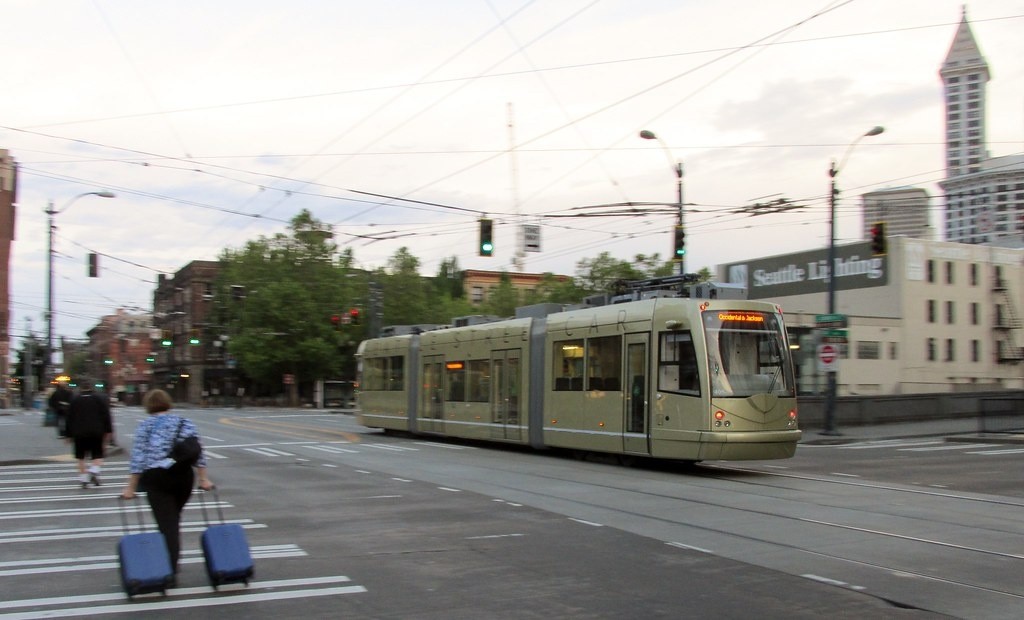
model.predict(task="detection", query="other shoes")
[90,477,100,486]
[81,482,88,489]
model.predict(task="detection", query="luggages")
[197,484,255,590]
[116,495,174,602]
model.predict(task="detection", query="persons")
[122,389,213,573]
[684,357,701,391]
[44,377,116,488]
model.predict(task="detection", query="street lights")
[42,190,115,392]
[639,130,686,276]
[820,124,884,439]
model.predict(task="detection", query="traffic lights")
[672,227,686,260]
[162,333,172,347]
[350,310,359,326]
[869,223,888,258]
[333,316,341,331]
[480,218,494,257]
[191,333,199,346]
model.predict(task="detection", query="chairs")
[556,375,620,391]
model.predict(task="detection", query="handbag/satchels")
[171,420,202,467]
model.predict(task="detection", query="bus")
[352,296,802,463]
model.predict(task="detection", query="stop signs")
[819,344,837,364]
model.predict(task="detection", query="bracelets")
[199,478,207,482]
